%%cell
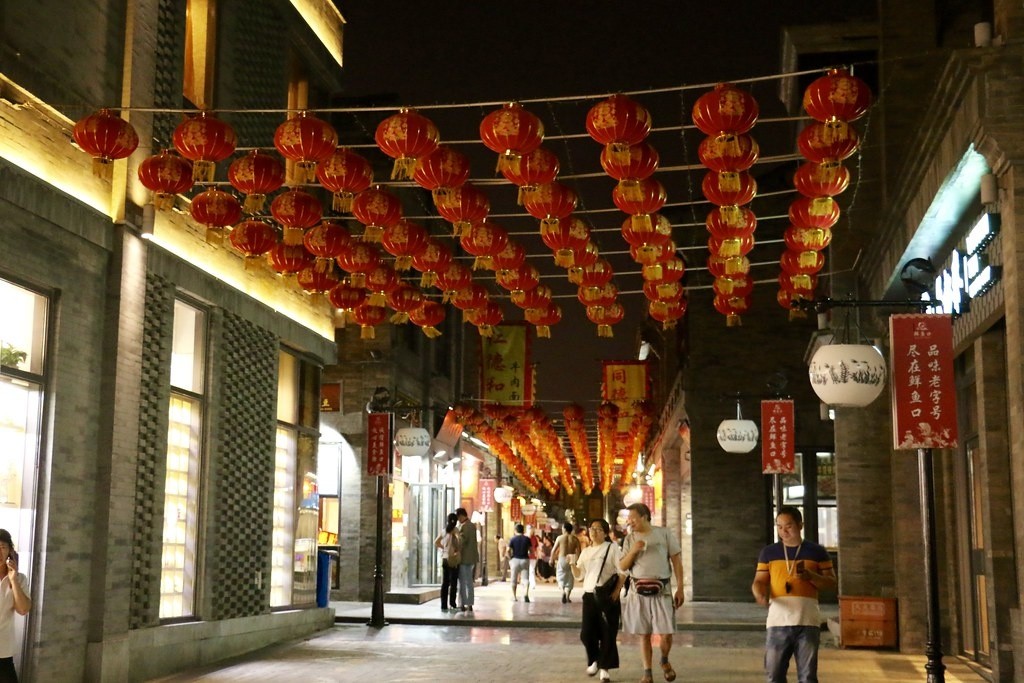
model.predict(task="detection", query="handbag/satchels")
[447,551,463,567]
[594,574,619,603]
[631,577,670,595]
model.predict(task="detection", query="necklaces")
[784,538,802,594]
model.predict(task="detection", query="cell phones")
[796,560,805,574]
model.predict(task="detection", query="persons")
[619,502,684,683]
[0,529,32,683]
[493,524,627,585]
[751,507,837,683]
[568,518,629,683]
[548,522,581,604]
[435,507,480,612]
[507,524,535,603]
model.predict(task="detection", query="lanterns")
[138,149,195,214]
[692,82,760,327]
[479,102,624,337]
[621,403,655,485]
[599,402,620,497]
[171,111,285,268]
[778,67,872,320]
[375,108,509,338]
[73,108,139,179]
[562,406,594,495]
[270,111,402,341]
[586,94,687,331]
[453,402,577,495]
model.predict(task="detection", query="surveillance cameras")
[366,401,379,413]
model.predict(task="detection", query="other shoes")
[660,661,676,682]
[450,607,459,612]
[467,605,472,611]
[600,668,609,680]
[587,661,599,674]
[525,596,529,602]
[566,599,571,603]
[460,606,466,611]
[562,593,566,603]
[640,674,653,683]
[442,608,448,612]
